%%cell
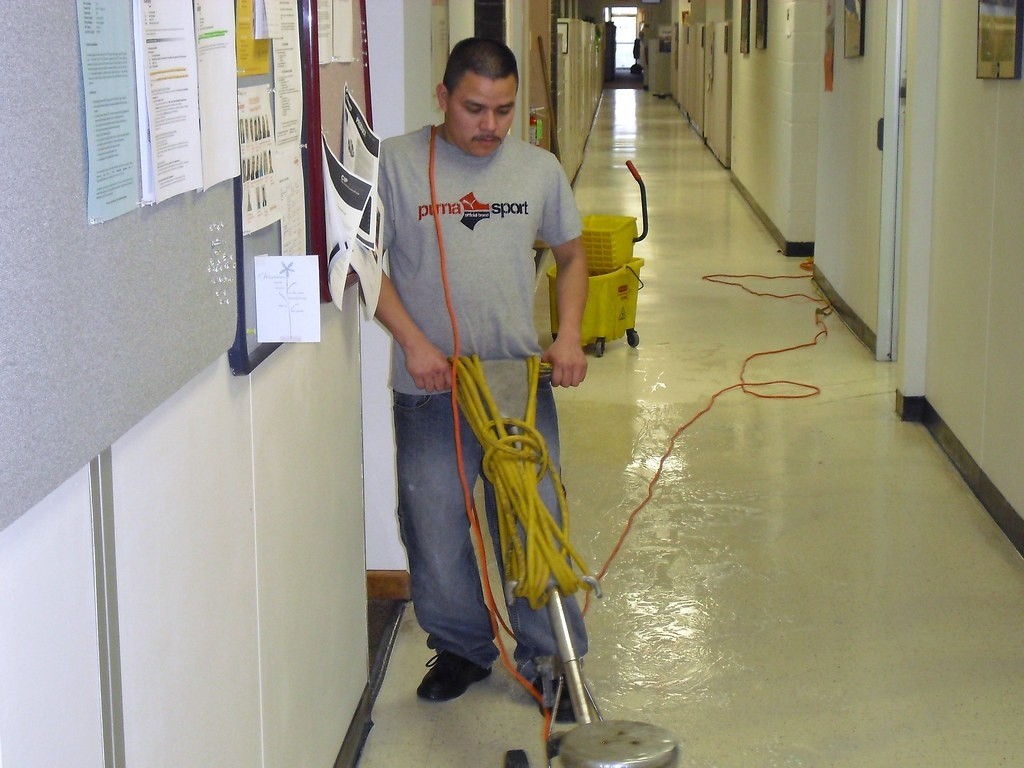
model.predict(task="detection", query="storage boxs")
[576,216,638,272]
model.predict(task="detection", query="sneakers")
[533,673,577,723]
[417,651,492,701]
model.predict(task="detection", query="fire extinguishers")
[529,105,545,147]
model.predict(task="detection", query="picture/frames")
[557,21,568,54]
[754,0,767,49]
[976,0,1024,80]
[843,0,866,59]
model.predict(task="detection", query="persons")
[360,37,590,723]
